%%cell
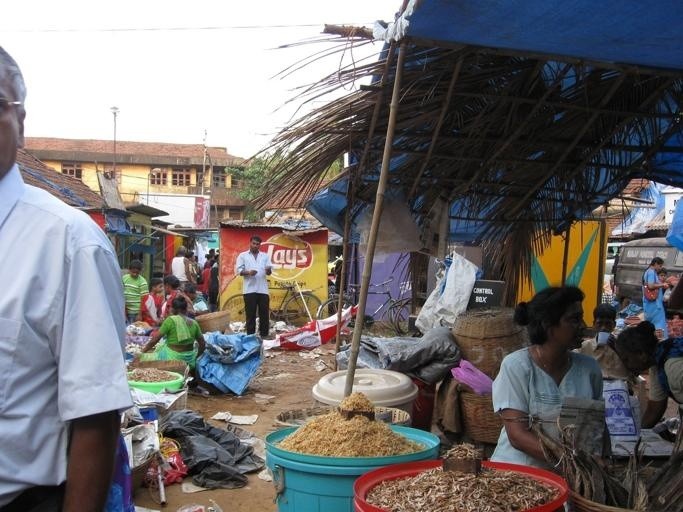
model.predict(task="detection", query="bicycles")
[221,281,322,327]
[388,298,410,327]
[316,276,408,331]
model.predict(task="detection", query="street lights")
[109,105,119,179]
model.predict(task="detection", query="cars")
[604,241,624,286]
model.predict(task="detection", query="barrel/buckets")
[264,423,442,511]
[312,368,419,417]
[353,459,569,511]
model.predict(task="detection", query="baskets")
[564,466,659,511]
[137,360,186,373]
[196,311,230,332]
[275,407,411,428]
[459,390,503,443]
[452,310,527,379]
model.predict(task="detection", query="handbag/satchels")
[644,283,658,301]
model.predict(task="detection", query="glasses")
[0,98,23,114]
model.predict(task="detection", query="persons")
[656,267,672,314]
[139,240,219,325]
[579,296,633,387]
[140,294,205,378]
[638,256,668,342]
[613,317,682,438]
[234,235,272,341]
[0,45,135,512]
[120,259,149,323]
[490,286,612,512]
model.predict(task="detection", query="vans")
[609,237,683,308]
